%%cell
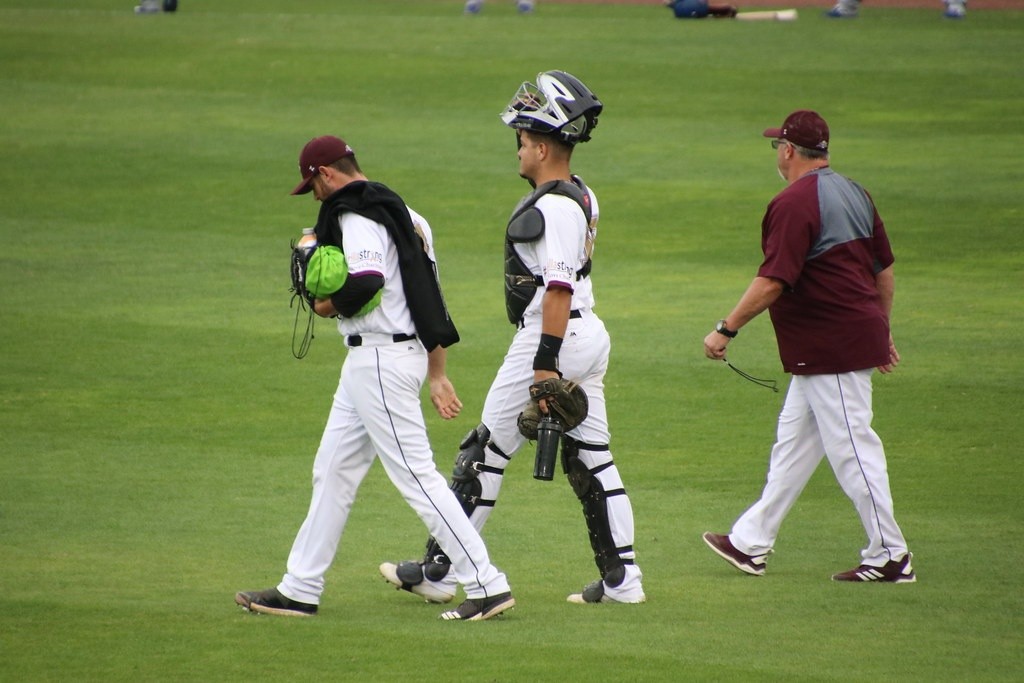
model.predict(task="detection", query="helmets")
[499,68,603,144]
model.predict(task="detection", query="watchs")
[715,319,740,338]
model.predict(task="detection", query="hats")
[764,110,830,151]
[289,136,354,195]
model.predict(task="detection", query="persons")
[703,109,916,585]
[379,70,646,604]
[825,0,967,18]
[235,135,515,621]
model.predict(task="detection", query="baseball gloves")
[517,377,590,440]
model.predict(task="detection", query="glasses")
[771,139,796,150]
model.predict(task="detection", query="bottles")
[533,416,563,481]
[296,227,318,282]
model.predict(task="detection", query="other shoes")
[826,5,859,18]
[945,5,967,18]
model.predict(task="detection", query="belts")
[346,333,416,345]
[515,310,582,332]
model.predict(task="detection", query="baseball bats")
[736,8,799,21]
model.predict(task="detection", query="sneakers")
[566,580,645,605]
[702,531,767,577]
[379,559,453,604]
[830,552,918,584]
[437,592,517,621]
[233,588,320,618]
